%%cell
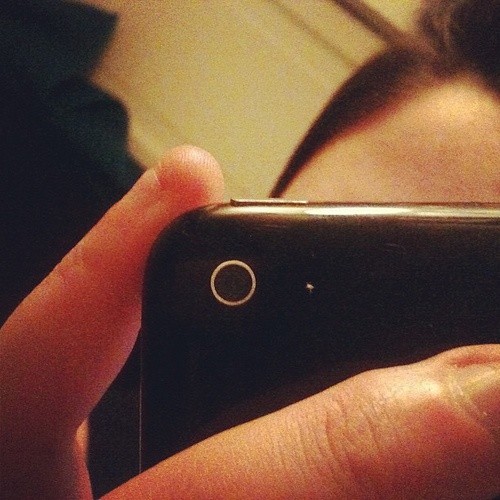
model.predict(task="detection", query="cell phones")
[142,194,500,476]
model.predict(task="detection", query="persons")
[1,0,499,497]
